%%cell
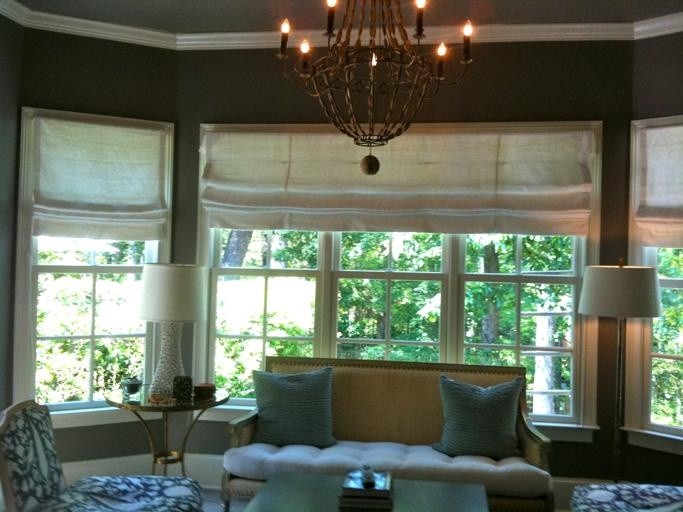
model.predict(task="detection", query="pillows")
[252,366,336,448]
[431,375,524,458]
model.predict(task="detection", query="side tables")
[105,389,230,476]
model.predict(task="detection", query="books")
[338,468,394,512]
[193,382,216,397]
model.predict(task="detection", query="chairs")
[570,483,683,512]
[0,399,203,512]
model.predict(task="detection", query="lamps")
[275,0,474,176]
[578,257,663,484]
[140,261,207,399]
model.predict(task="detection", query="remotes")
[361,464,375,489]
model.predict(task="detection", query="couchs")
[220,357,555,512]
